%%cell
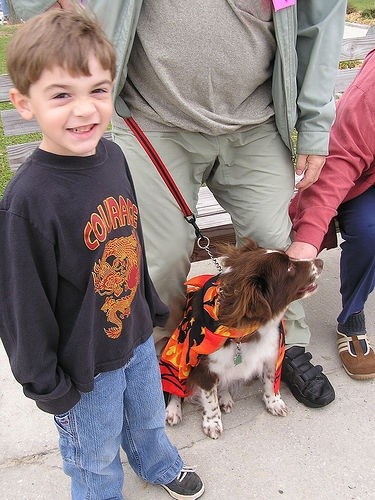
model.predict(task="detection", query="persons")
[0,10,205,500]
[4,1,347,409]
[287,43,375,380]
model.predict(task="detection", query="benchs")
[0,34,375,262]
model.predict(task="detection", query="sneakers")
[281,344,336,408]
[161,467,204,500]
[154,338,186,408]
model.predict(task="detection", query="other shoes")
[335,323,375,380]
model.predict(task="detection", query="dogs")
[158,235,325,442]
